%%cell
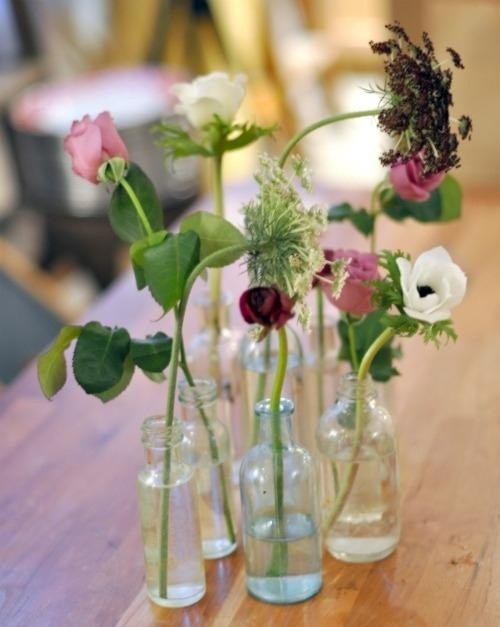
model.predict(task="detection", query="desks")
[0,187,500,627]
